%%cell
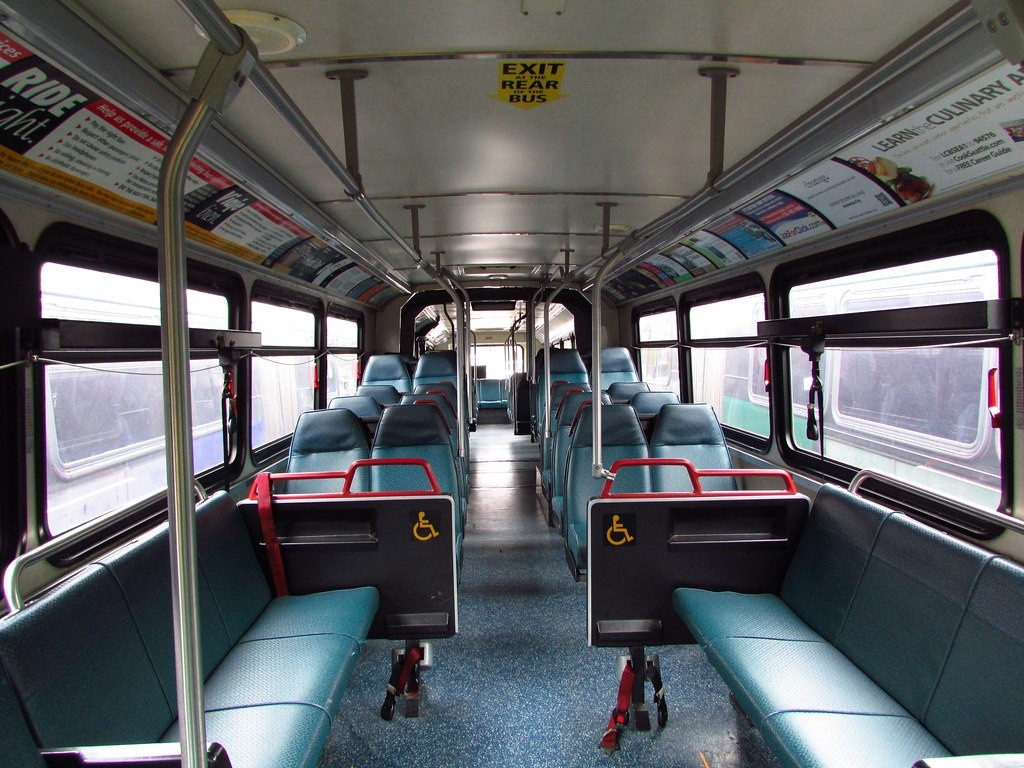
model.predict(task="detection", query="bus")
[42,262,358,536]
[641,249,1002,511]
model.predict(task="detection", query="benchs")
[236,458,460,717]
[0,480,383,768]
[664,468,1024,768]
[585,458,814,731]
[284,344,737,582]
